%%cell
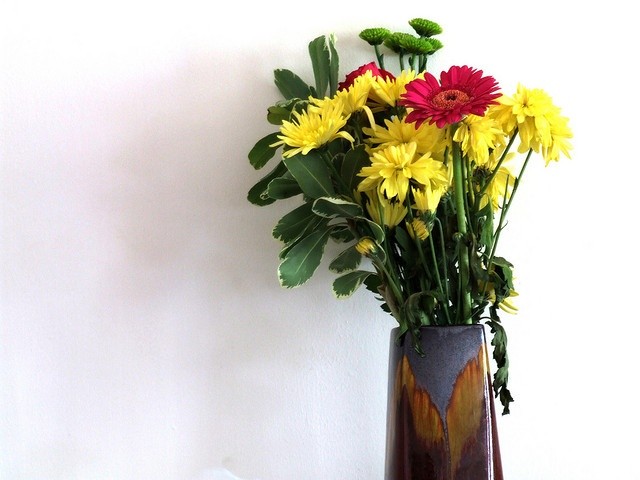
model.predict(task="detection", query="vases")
[383,323,504,480]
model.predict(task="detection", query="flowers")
[246,18,573,416]
